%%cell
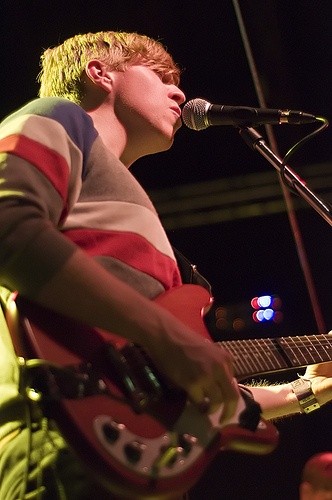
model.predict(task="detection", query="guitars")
[4,287,332,497]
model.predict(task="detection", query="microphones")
[182,98,317,131]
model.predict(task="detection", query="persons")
[0,31,332,500]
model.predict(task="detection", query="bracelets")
[290,378,321,415]
